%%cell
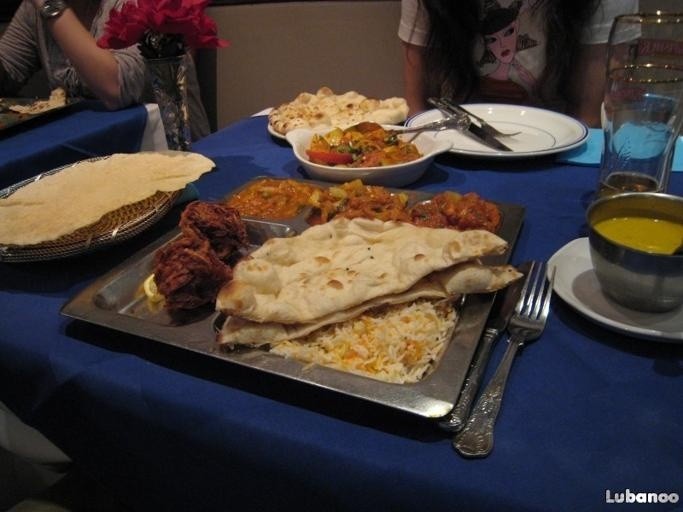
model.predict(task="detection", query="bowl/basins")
[286,124,452,190]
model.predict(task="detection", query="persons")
[0,1,210,145]
[395,4,640,129]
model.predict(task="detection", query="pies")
[214,218,524,347]
[269,87,408,134]
[9,88,65,114]
[0,149,217,247]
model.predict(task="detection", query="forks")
[437,260,557,457]
[444,93,521,138]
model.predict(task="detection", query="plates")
[405,104,589,156]
[546,234,682,343]
[267,121,285,145]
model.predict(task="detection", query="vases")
[136,51,196,154]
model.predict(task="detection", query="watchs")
[36,1,68,20]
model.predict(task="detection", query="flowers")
[91,0,232,151]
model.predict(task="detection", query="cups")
[585,192,682,311]
[598,10,683,199]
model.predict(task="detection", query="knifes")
[427,96,509,151]
